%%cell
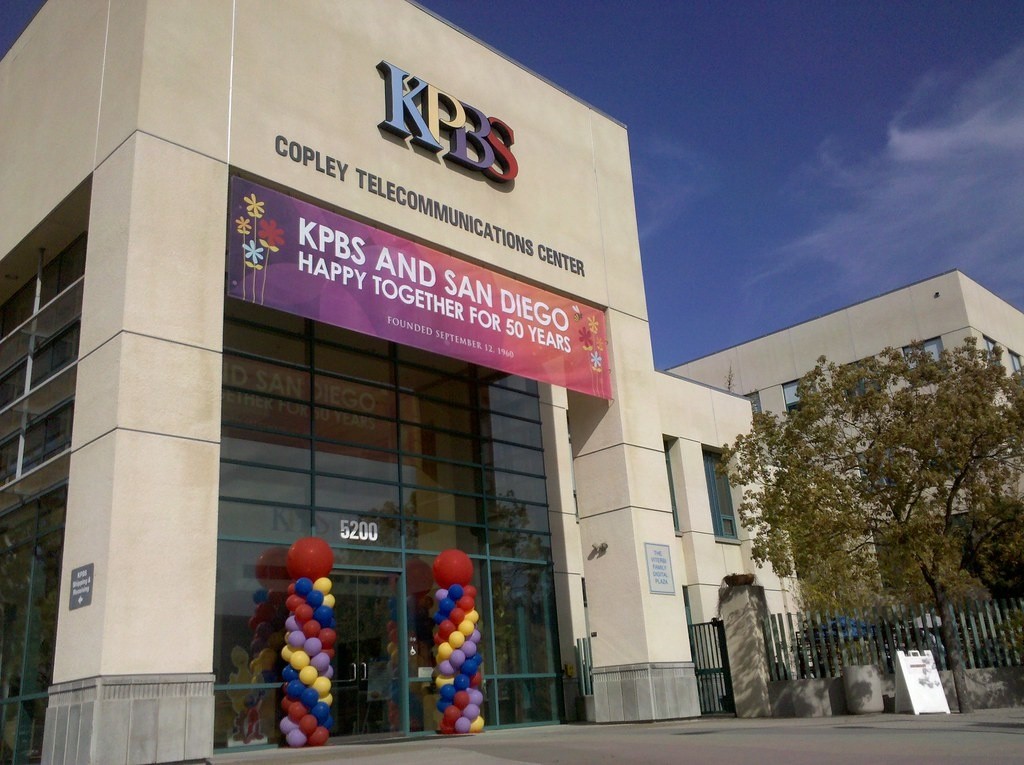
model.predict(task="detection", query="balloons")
[387,559,435,733]
[279,537,335,747]
[248,547,290,684]
[433,549,485,734]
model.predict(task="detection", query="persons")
[5,660,52,721]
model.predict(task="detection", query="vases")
[725,574,755,586]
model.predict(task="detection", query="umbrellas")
[805,613,960,642]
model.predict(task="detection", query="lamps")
[592,543,608,552]
